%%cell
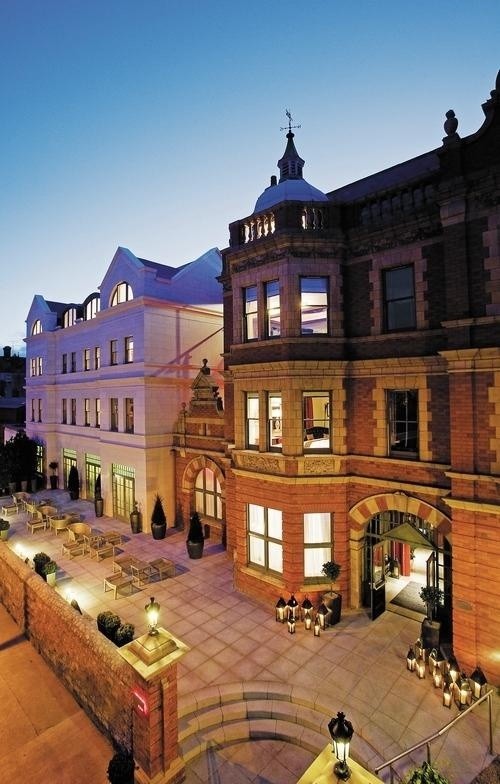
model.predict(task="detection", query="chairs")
[0,491,177,600]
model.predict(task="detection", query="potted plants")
[319,560,343,627]
[66,464,80,501]
[186,511,205,560]
[49,462,59,489]
[97,611,135,647]
[418,585,448,662]
